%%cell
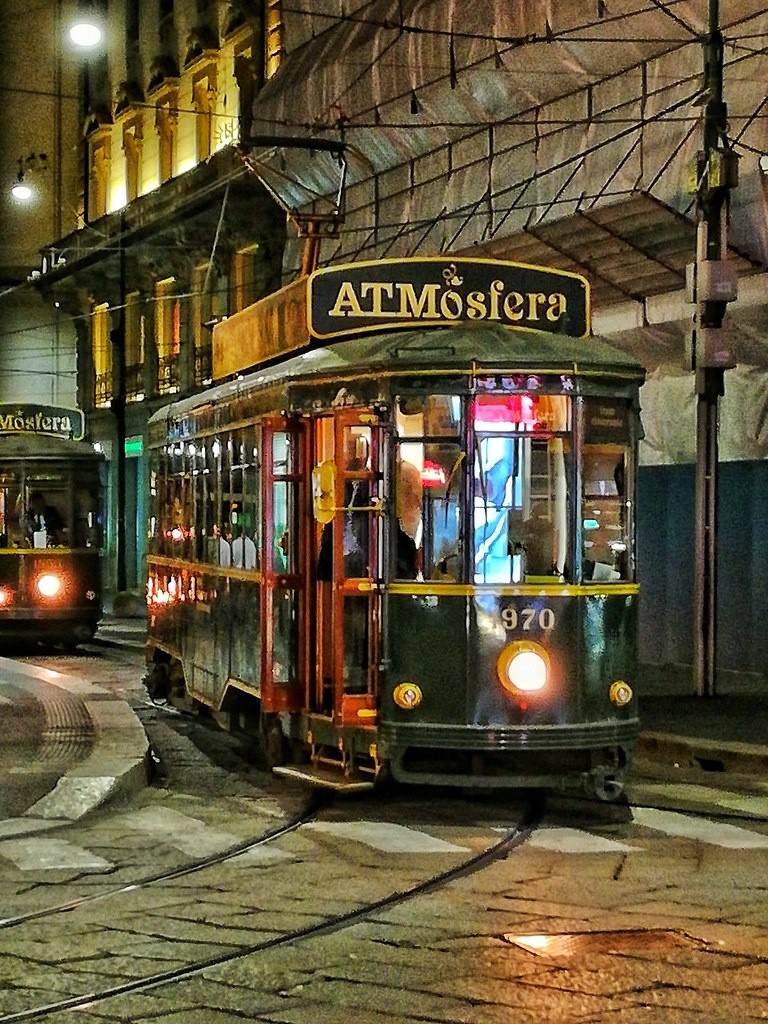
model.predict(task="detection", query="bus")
[0,402,107,651]
[139,252,645,803]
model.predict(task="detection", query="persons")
[26,491,69,545]
